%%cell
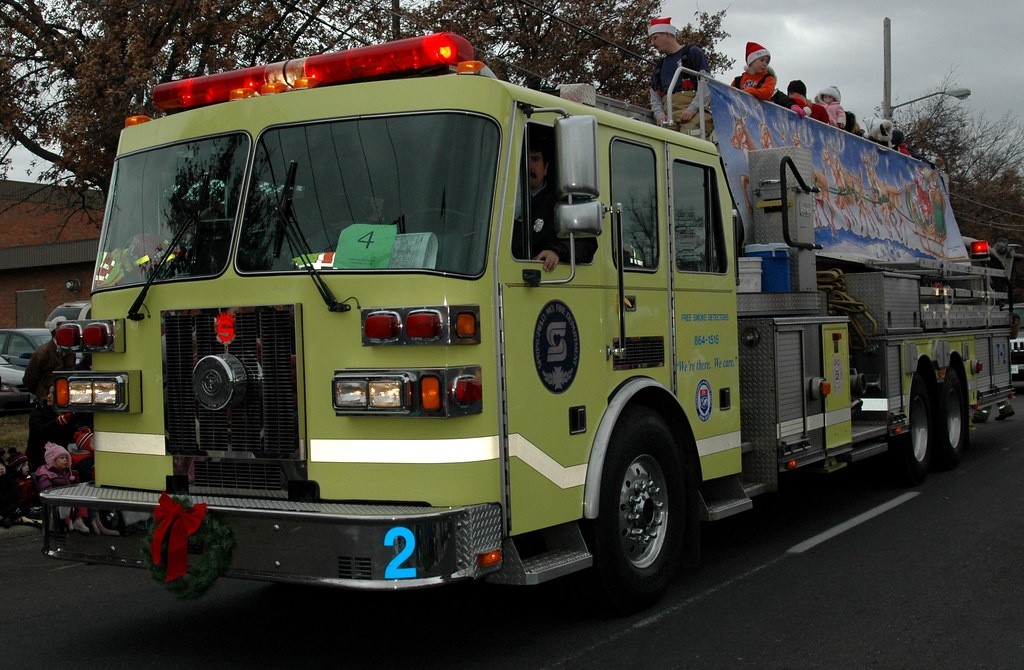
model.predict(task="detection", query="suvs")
[44,299,92,338]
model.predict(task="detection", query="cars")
[0,328,52,366]
[0,354,38,416]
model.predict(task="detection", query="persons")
[817,85,847,129]
[786,80,830,125]
[0,447,44,528]
[730,41,775,101]
[69,430,95,484]
[843,111,865,137]
[24,382,94,474]
[867,119,935,170]
[649,16,714,139]
[22,314,77,406]
[767,67,805,118]
[35,441,90,534]
[509,139,571,273]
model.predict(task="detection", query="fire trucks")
[34,33,1018,622]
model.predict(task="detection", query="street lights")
[883,85,973,121]
